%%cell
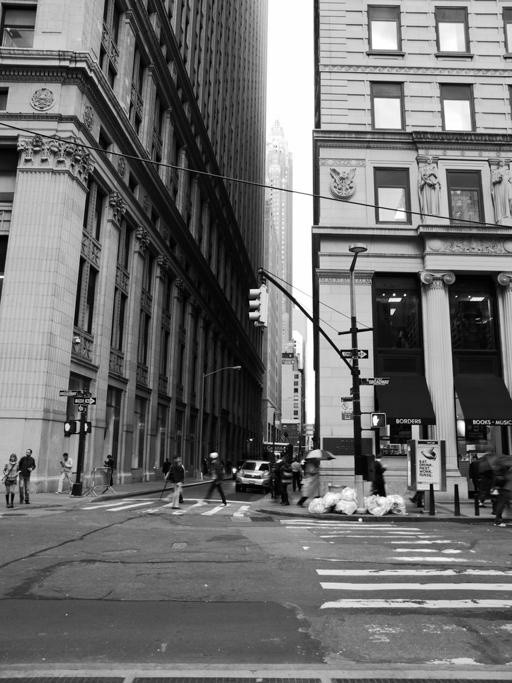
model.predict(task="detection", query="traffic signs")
[360,377,390,385]
[341,349,368,358]
[74,397,96,404]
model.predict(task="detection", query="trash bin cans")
[327,484,346,492]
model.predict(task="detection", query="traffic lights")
[63,420,76,436]
[370,412,386,428]
[248,288,260,320]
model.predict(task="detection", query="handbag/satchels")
[1,475,7,484]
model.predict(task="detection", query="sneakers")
[20,500,31,504]
[219,503,225,506]
[55,492,59,494]
[409,497,424,508]
[493,522,506,528]
[279,501,287,506]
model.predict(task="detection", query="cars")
[235,459,273,494]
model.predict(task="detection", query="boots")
[6,492,14,508]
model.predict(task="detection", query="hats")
[210,452,219,459]
[275,459,283,464]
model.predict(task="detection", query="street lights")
[348,243,368,514]
[196,365,242,481]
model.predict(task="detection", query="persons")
[369,453,386,496]
[104,455,114,487]
[468,456,482,494]
[202,457,209,478]
[3,453,21,509]
[18,449,37,505]
[168,456,186,509]
[163,458,171,476]
[280,458,290,507]
[494,463,512,528]
[56,453,77,495]
[411,491,426,507]
[206,452,230,509]
[291,457,302,492]
